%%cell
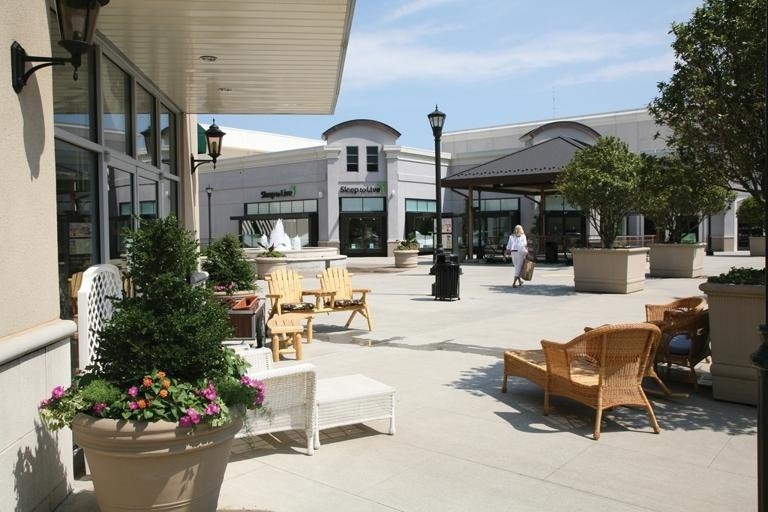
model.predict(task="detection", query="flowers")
[41,214,272,447]
[201,230,258,294]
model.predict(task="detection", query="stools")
[266,318,305,362]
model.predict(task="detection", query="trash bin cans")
[434,254,460,302]
[453,244,465,263]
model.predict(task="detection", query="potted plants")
[393,232,420,270]
[258,244,288,281]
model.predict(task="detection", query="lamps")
[9,0,108,96]
[189,117,226,178]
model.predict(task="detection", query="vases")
[72,413,243,512]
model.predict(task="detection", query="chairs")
[264,266,373,343]
[501,322,662,440]
[583,294,712,393]
[173,346,397,457]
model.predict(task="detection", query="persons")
[504,225,534,288]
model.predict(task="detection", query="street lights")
[205,184,214,260]
[426,106,447,274]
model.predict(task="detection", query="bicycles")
[481,236,515,262]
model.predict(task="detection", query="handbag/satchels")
[521,259,536,281]
